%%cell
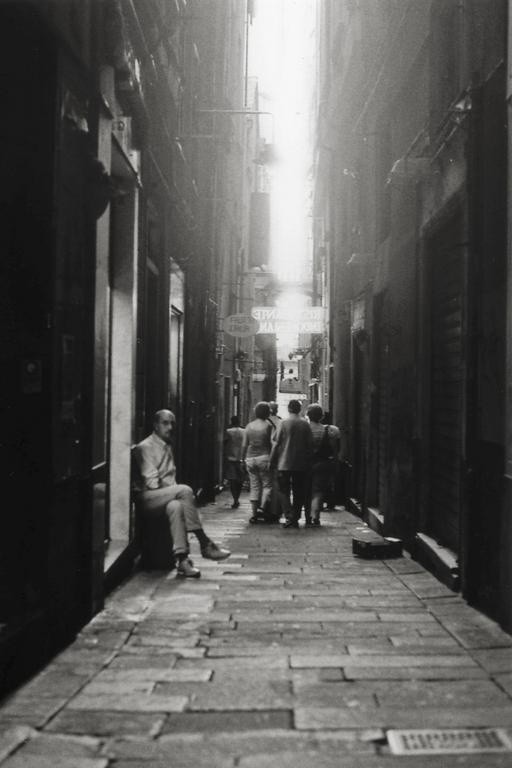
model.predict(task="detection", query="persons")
[130,408,230,576]
[223,398,342,527]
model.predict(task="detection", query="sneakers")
[200,539,231,560]
[175,558,201,576]
[247,510,321,528]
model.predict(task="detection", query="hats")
[254,402,270,418]
[288,400,301,412]
[306,404,323,417]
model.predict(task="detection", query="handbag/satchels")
[320,457,343,491]
